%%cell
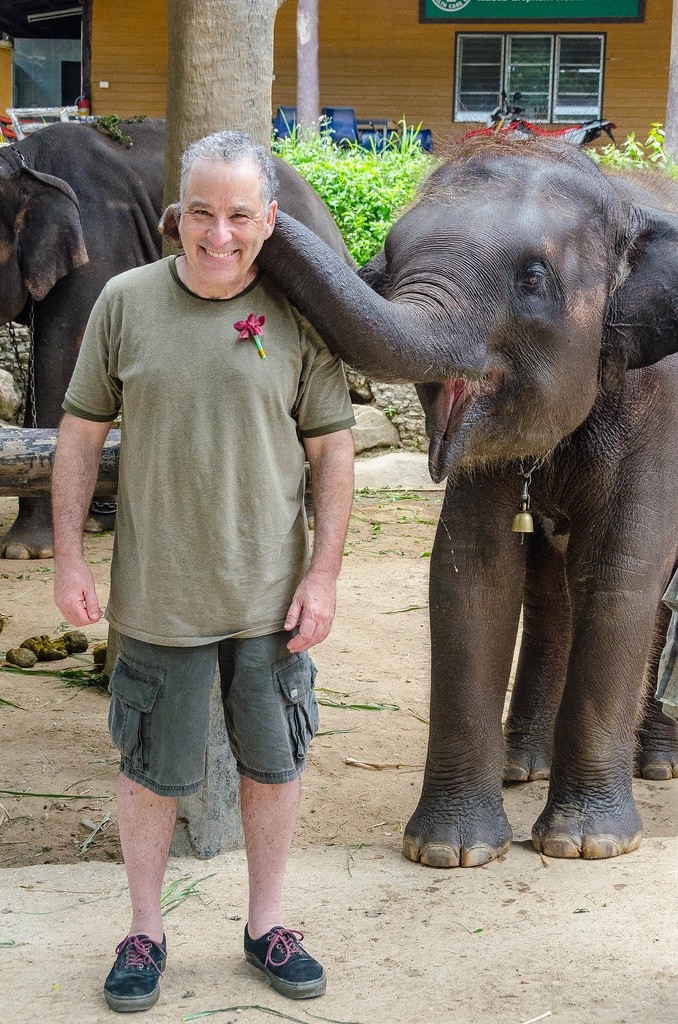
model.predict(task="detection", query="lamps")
[27,6,84,23]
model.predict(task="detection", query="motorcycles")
[458,90,617,148]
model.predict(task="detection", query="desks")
[353,118,389,156]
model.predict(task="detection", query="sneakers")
[243,922,327,999]
[104,931,167,1012]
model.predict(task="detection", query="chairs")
[272,105,434,155]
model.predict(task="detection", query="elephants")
[157,137,678,870]
[0,120,359,561]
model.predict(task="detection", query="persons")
[49,129,359,1013]
